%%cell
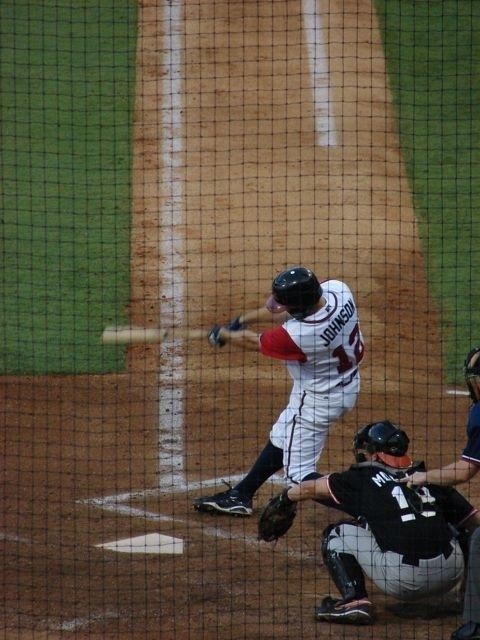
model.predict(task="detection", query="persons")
[253,420,479,624]
[194,265,366,517]
[392,348,480,639]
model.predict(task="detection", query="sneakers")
[311,594,376,626]
[193,483,255,518]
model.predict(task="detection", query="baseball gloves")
[257,486,297,542]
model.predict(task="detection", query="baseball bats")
[103,328,210,345]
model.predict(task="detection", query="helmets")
[348,420,413,472]
[261,265,323,315]
[463,346,480,405]
